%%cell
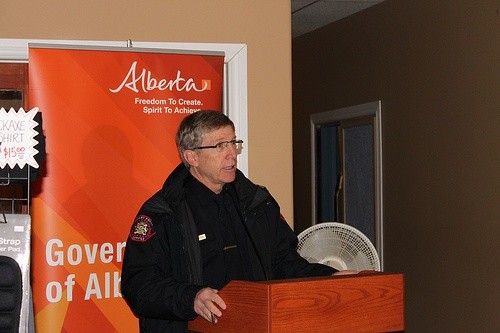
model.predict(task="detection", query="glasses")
[191,140,243,154]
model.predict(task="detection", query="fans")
[297,222,380,272]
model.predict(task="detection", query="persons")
[121,109,359,333]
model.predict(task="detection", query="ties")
[210,190,245,285]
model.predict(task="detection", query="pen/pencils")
[211,312,216,328]
